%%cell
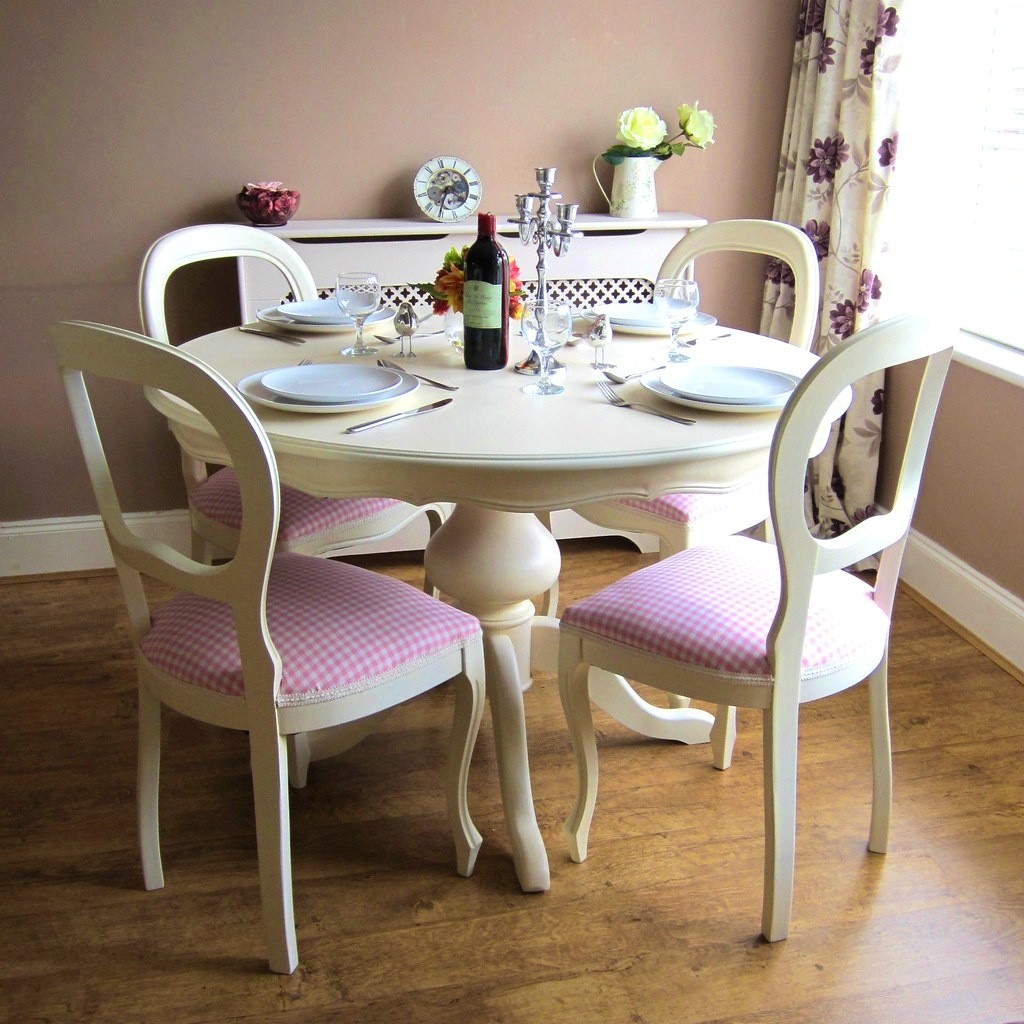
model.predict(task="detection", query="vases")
[444,307,464,354]
[593,152,663,218]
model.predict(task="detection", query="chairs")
[48,220,959,975]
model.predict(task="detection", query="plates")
[639,364,802,414]
[257,300,397,332]
[237,364,420,413]
[581,298,717,337]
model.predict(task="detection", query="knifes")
[347,399,455,434]
[239,327,305,345]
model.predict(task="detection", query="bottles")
[463,213,510,369]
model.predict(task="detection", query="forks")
[596,380,697,426]
[299,358,315,365]
[675,333,732,349]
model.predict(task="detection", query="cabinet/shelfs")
[237,211,707,560]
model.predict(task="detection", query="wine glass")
[654,278,699,362]
[337,273,382,357]
[521,299,573,395]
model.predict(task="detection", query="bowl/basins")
[237,192,301,226]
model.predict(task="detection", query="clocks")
[413,156,482,223]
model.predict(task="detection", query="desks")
[144,320,852,892]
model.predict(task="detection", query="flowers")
[407,245,526,320]
[601,100,716,166]
[237,182,300,224]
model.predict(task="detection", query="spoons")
[373,312,446,344]
[602,364,666,384]
[377,358,459,390]
